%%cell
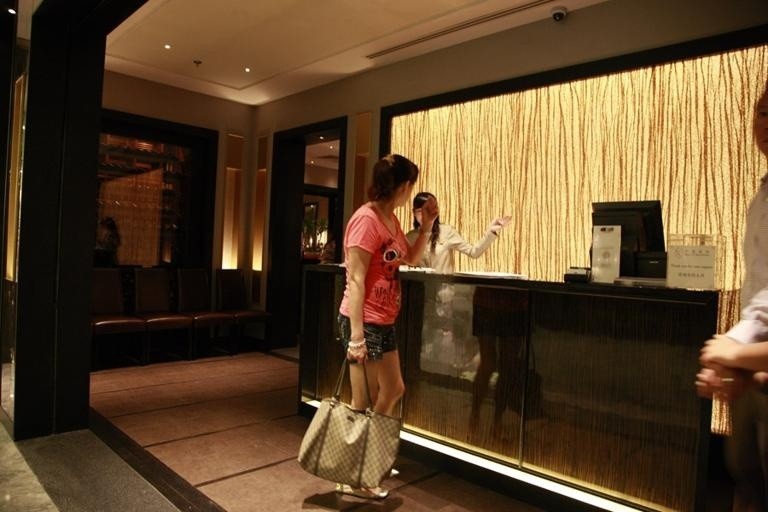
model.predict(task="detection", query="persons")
[466,287,528,443]
[399,191,512,270]
[319,225,336,264]
[95,215,122,268]
[726,86,768,511]
[697,283,768,400]
[338,153,441,498]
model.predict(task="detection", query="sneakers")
[335,481,390,499]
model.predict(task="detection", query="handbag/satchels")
[298,352,401,490]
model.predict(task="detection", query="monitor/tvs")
[592,199,665,253]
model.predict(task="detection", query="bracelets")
[489,229,499,237]
[420,228,433,236]
[348,338,366,348]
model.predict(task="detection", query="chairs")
[94,262,271,367]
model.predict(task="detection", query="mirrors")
[99,115,217,266]
